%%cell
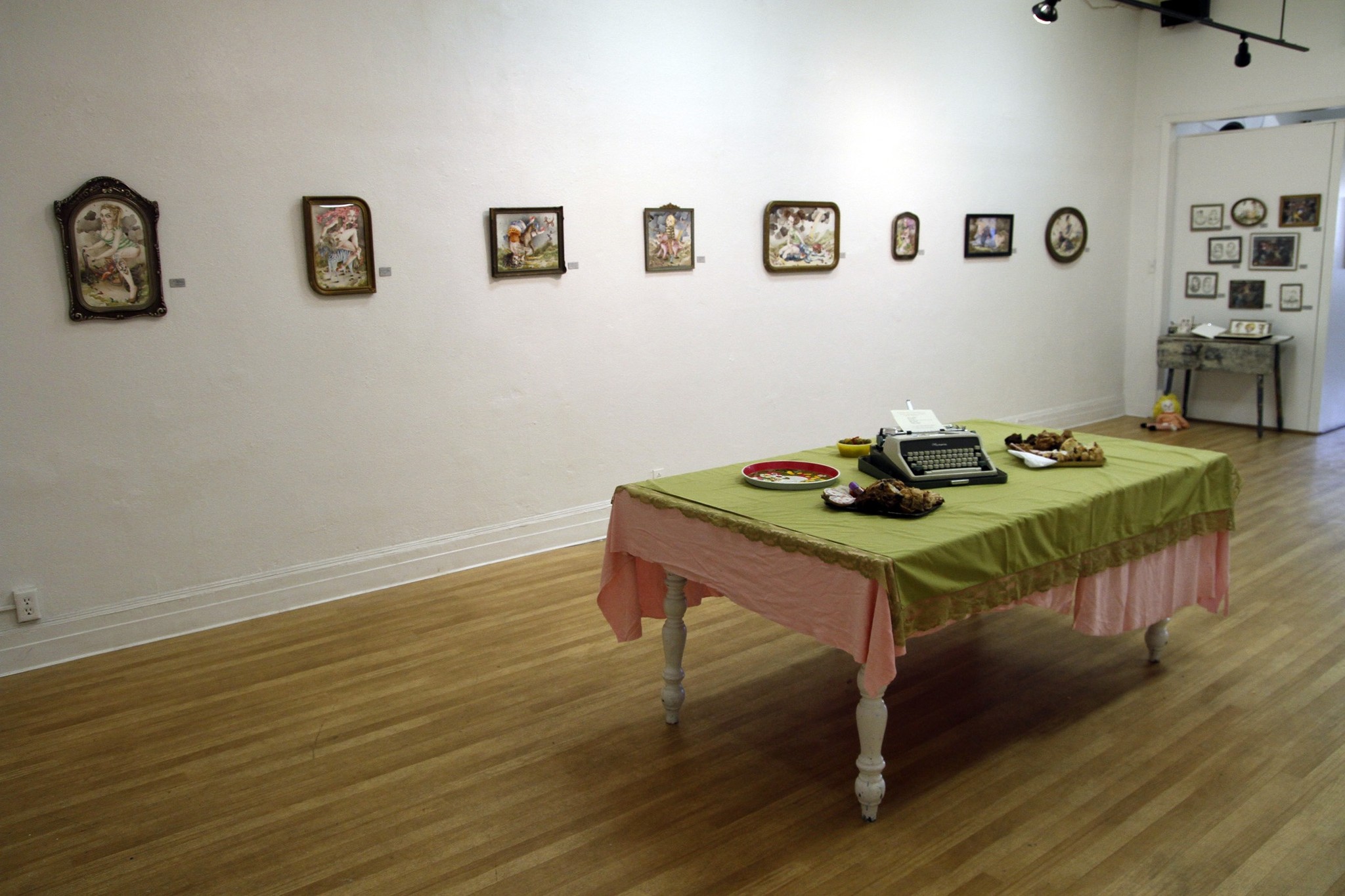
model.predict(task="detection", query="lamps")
[1234,30,1253,68]
[1030,0,1062,24]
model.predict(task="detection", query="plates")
[1050,456,1106,467]
[741,461,841,491]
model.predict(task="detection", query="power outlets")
[11,586,42,626]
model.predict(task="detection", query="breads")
[854,478,945,513]
[1004,429,1103,461]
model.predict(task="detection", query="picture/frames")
[1278,283,1303,310]
[488,206,568,278]
[1249,232,1300,271]
[1230,320,1272,334]
[962,212,1014,258]
[1184,272,1219,299]
[891,211,921,261]
[1190,204,1223,231]
[1278,194,1321,227]
[1046,205,1090,264]
[1231,197,1268,226]
[761,200,839,272]
[1227,279,1265,310]
[1208,235,1244,264]
[303,194,378,296]
[644,204,696,272]
[52,175,168,325]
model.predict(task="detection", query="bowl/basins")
[837,441,873,458]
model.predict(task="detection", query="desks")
[1155,334,1286,439]
[594,418,1242,818]
